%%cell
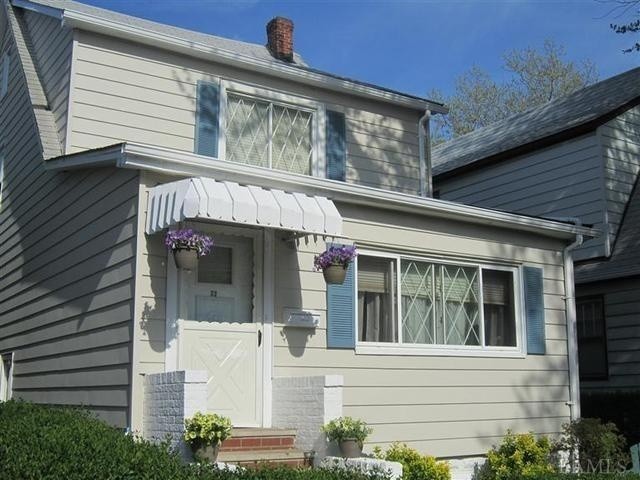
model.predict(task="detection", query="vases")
[322,263,347,285]
[173,246,198,270]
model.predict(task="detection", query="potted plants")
[319,416,375,458]
[182,410,234,464]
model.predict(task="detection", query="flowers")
[164,227,214,259]
[312,241,359,273]
